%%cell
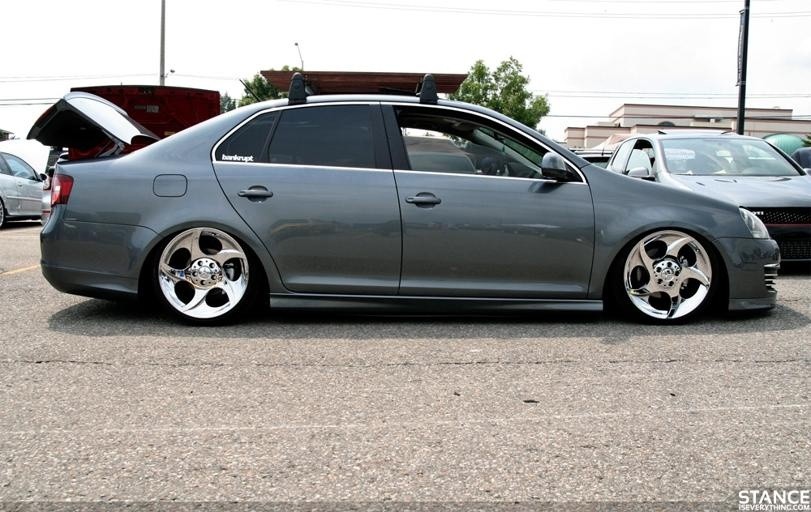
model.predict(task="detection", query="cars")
[0,152,51,229]
[64,85,221,158]
[566,128,810,278]
[39,152,67,224]
[26,71,785,327]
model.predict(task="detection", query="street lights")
[290,43,307,72]
[158,65,174,85]
